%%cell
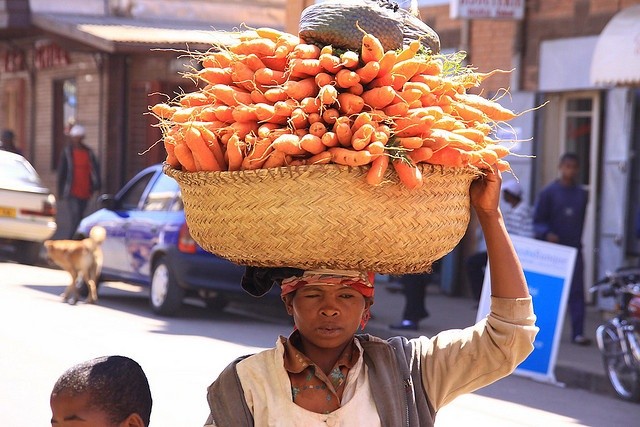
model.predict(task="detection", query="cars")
[1,147,56,265]
[75,162,282,314]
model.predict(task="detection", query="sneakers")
[573,336,590,345]
[390,320,418,330]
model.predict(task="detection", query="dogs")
[43,225,107,305]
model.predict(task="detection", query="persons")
[55,124,104,243]
[499,179,534,239]
[0,131,19,153]
[205,164,540,427]
[532,153,594,347]
[388,265,429,331]
[465,202,492,309]
[50,356,152,427]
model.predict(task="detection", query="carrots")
[138,21,550,189]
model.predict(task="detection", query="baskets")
[162,161,487,275]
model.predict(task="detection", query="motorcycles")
[588,267,640,399]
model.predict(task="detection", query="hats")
[501,181,522,198]
[70,125,84,137]
